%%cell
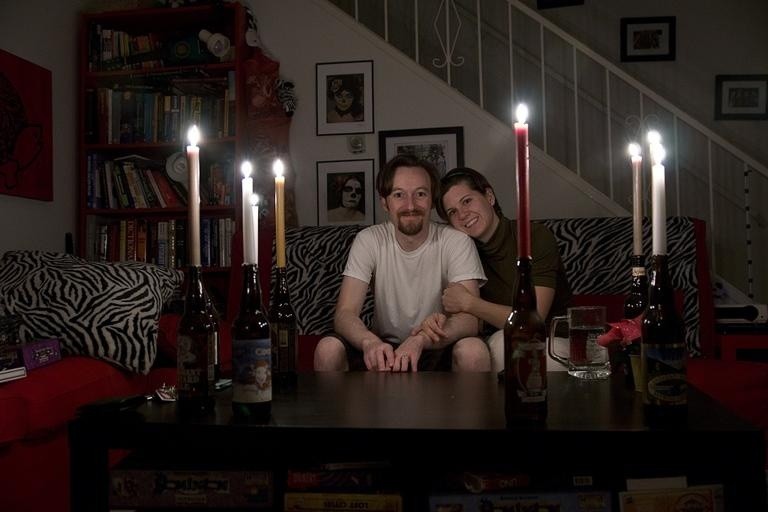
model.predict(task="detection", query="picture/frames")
[536,1,585,10]
[378,126,464,224]
[714,74,768,121]
[316,60,374,137]
[619,16,677,61]
[316,158,376,228]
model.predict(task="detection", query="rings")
[401,351,410,359]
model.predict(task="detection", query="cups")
[548,304,611,379]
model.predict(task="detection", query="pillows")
[0,246,180,377]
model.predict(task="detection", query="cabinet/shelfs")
[75,4,280,325]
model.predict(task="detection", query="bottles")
[504,255,547,423]
[268,267,296,388]
[624,253,655,384]
[231,264,272,419]
[641,254,688,404]
[176,266,218,414]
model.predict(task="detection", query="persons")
[327,75,356,121]
[326,174,365,222]
[312,152,493,374]
[410,165,589,373]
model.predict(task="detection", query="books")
[85,153,235,209]
[0,313,28,384]
[99,27,166,72]
[85,213,237,273]
[93,84,236,146]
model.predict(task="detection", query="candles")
[514,103,531,256]
[627,142,644,255]
[272,159,286,266]
[648,129,661,164]
[185,124,202,267]
[251,191,260,262]
[649,146,669,255]
[240,160,254,264]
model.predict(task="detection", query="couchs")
[264,215,713,434]
[0,248,232,511]
